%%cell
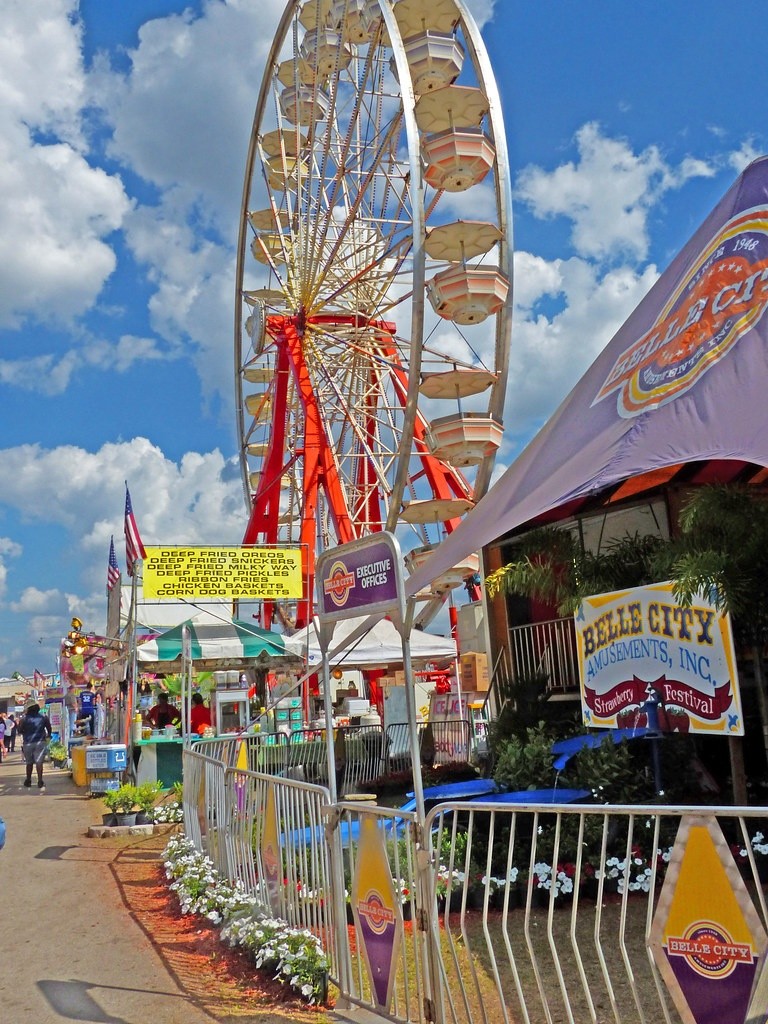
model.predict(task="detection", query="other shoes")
[38,781,44,787]
[11,749,15,752]
[24,779,31,787]
[3,750,6,756]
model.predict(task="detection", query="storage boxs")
[377,677,395,688]
[388,662,425,671]
[86,744,127,771]
[387,670,415,685]
[460,651,489,692]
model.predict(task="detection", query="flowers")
[160,833,332,997]
[154,802,183,825]
[392,831,768,904]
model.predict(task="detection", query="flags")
[123,481,148,578]
[107,535,121,597]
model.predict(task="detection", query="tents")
[129,147,768,763]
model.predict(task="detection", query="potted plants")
[49,742,67,767]
[133,779,163,825]
[116,782,137,826]
[102,789,122,828]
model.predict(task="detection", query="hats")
[24,699,38,710]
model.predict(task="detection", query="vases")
[517,888,539,909]
[605,877,622,895]
[442,892,461,913]
[402,900,416,921]
[492,890,518,911]
[300,971,328,1005]
[564,883,583,903]
[582,879,602,900]
[756,861,768,884]
[466,892,482,908]
[541,889,563,909]
[346,903,354,926]
[737,861,753,880]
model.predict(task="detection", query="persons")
[0,680,106,788]
[144,694,181,736]
[190,693,215,739]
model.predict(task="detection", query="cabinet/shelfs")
[86,770,124,798]
